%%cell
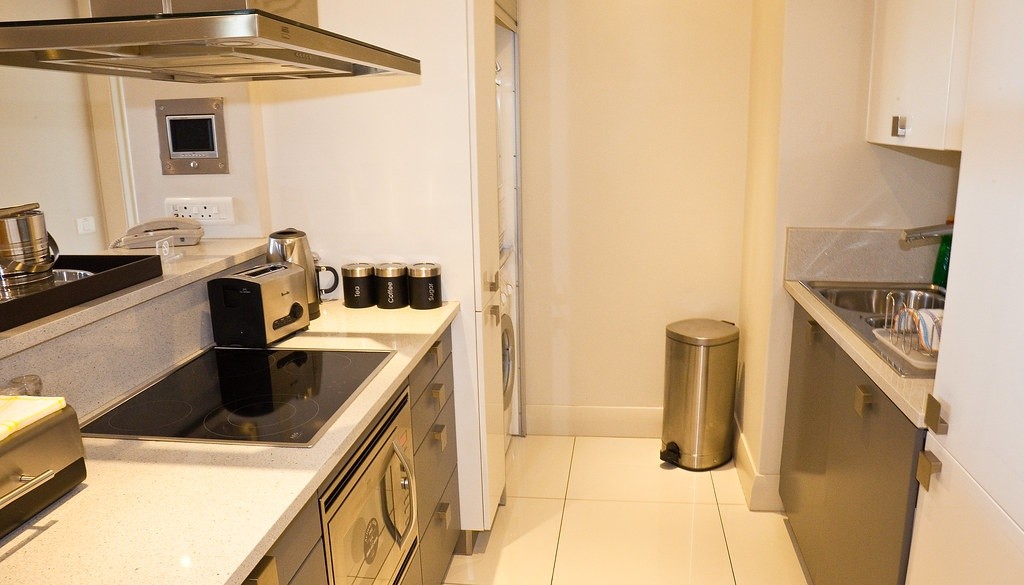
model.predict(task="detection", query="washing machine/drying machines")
[496,246,521,460]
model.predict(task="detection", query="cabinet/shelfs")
[236,490,329,585]
[775,300,927,584]
[904,0,1022,585]
[883,288,946,360]
[257,0,508,556]
[861,1,975,154]
[408,325,464,585]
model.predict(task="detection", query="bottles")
[932,213,954,295]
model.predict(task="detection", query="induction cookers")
[78,346,399,449]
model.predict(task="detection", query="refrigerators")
[264,0,506,558]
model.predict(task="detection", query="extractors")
[0,0,421,84]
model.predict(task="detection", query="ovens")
[317,385,420,585]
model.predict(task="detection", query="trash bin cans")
[660,318,740,472]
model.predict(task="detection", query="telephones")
[122,217,204,249]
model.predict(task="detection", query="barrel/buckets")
[0,201,60,286]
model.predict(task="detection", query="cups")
[340,262,375,308]
[311,257,339,303]
[409,263,441,309]
[374,263,410,308]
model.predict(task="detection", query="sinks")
[798,279,947,315]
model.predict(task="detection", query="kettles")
[267,227,322,322]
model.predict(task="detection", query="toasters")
[207,260,310,349]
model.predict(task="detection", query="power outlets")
[77,215,97,235]
[162,196,239,225]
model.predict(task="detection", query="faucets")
[898,222,955,252]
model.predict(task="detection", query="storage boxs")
[341,261,376,309]
[407,262,441,310]
[374,261,408,310]
